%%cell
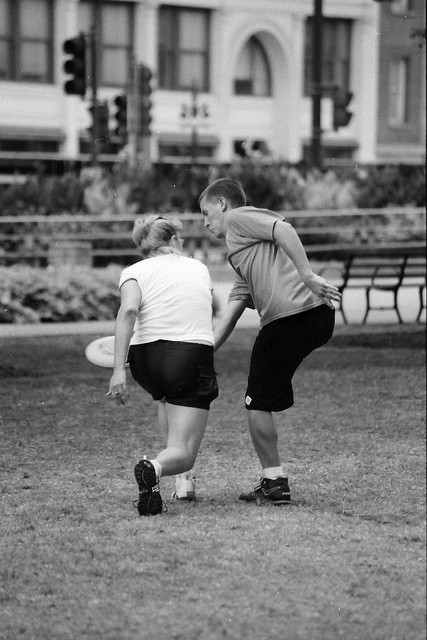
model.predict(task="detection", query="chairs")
[311,242,426,329]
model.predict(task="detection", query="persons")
[105,211,221,518]
[196,177,343,506]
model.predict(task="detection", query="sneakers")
[134,460,163,516]
[239,476,291,505]
[171,479,195,500]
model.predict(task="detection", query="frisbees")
[85,334,130,369]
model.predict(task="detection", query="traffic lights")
[333,87,352,127]
[113,97,128,140]
[62,32,89,95]
